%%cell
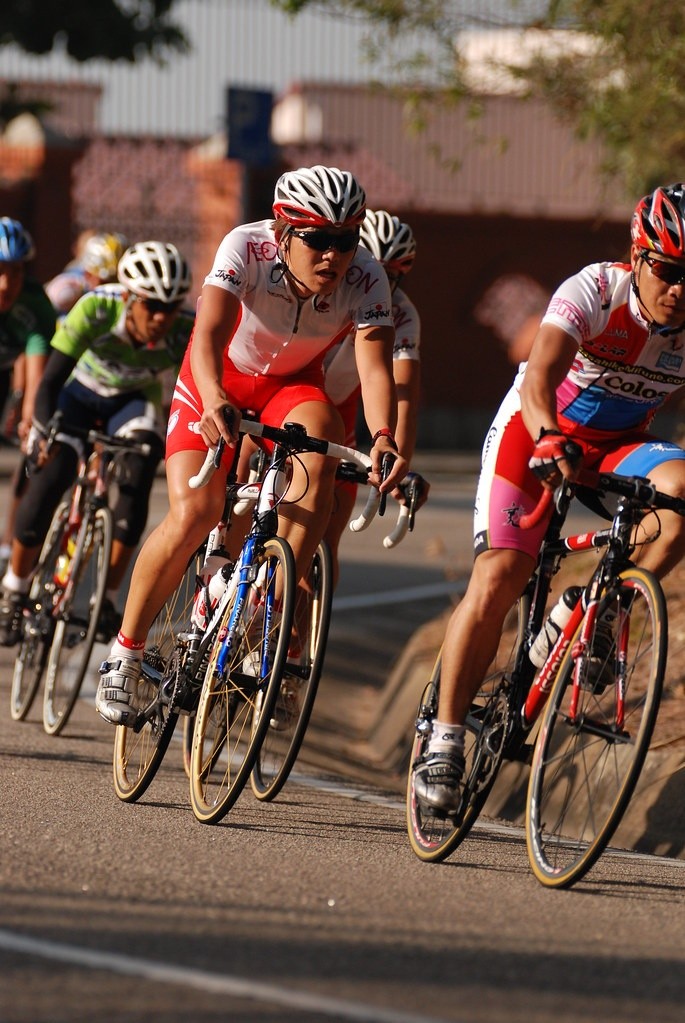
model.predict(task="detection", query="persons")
[412,182,685,811]
[226,209,430,732]
[95,165,409,725]
[0,232,131,577]
[0,217,56,437]
[0,241,196,647]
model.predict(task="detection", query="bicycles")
[0,396,429,824]
[404,442,685,888]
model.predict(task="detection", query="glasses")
[130,294,182,315]
[640,253,685,287]
[287,227,359,252]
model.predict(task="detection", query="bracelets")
[370,428,398,450]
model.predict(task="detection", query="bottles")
[528,585,586,668]
[53,519,96,588]
[247,555,278,625]
[190,563,234,631]
[194,545,233,604]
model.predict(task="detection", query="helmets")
[273,165,366,234]
[85,232,130,279]
[116,240,188,304]
[359,208,416,273]
[0,217,36,261]
[630,183,685,259]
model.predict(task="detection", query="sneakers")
[0,582,29,646]
[413,750,464,811]
[243,618,278,679]
[270,673,305,732]
[96,658,142,728]
[582,625,616,694]
